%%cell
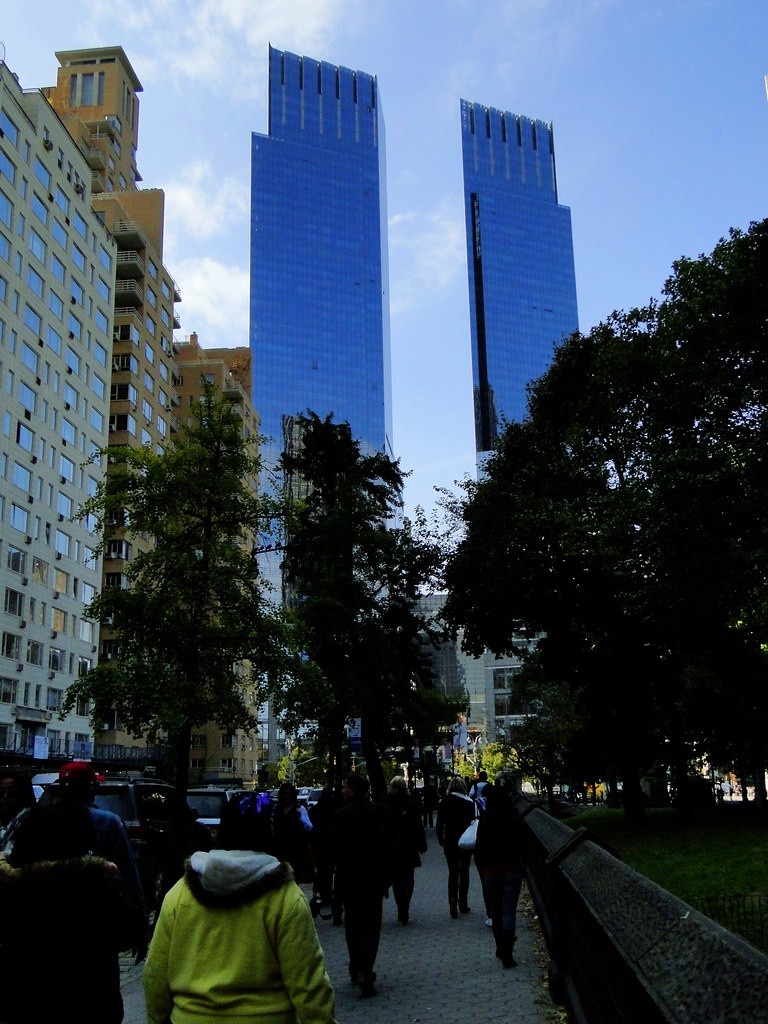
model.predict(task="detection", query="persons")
[0,760,535,1003]
[143,809,339,1024]
[0,804,134,1024]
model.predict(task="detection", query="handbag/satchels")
[458,802,480,850]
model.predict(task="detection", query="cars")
[0,757,342,895]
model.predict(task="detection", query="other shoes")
[459,906,470,913]
[358,977,377,996]
[451,909,458,918]
[485,918,493,926]
[496,949,502,957]
[430,824,433,827]
[503,954,517,967]
[352,972,376,985]
[333,911,343,926]
[424,823,427,827]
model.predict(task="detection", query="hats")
[59,761,93,783]
